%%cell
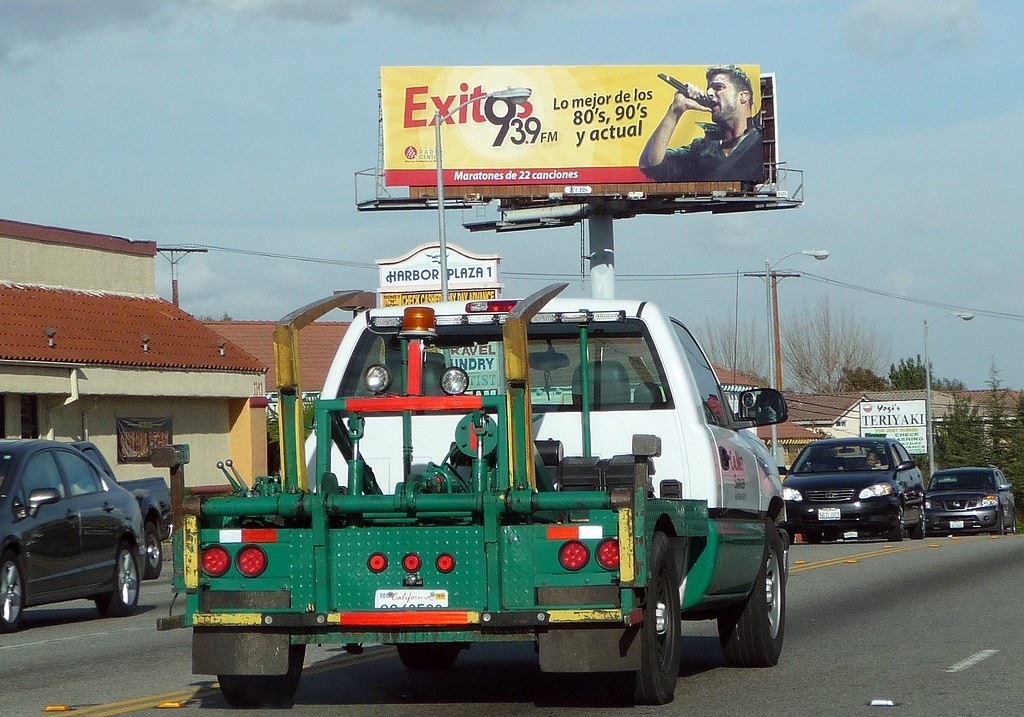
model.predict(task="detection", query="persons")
[863,451,882,468]
[754,393,777,426]
[638,66,763,181]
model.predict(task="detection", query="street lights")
[764,247,831,460]
[433,84,534,306]
[922,312,975,470]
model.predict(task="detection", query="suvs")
[921,464,1017,534]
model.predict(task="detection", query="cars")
[0,432,148,630]
[781,434,925,541]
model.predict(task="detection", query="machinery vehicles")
[154,282,791,707]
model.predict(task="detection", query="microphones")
[657,72,717,108]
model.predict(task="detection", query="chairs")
[811,449,837,470]
[572,361,631,405]
[423,350,448,398]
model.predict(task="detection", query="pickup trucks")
[65,439,172,578]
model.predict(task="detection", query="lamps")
[43,327,57,346]
[218,341,227,357]
[142,332,151,351]
